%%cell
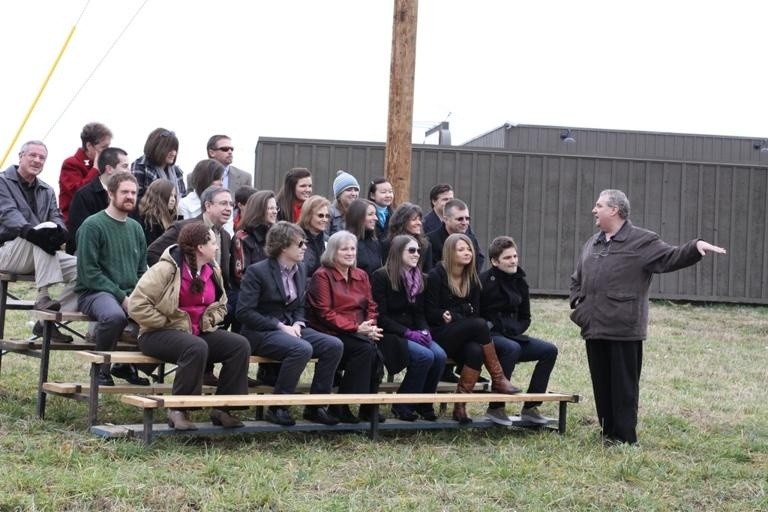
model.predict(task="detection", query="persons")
[571,189,727,447]
[57,122,557,429]
[0,140,79,343]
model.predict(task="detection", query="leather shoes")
[29,295,76,345]
[191,362,265,387]
[90,364,151,389]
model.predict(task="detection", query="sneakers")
[485,407,513,428]
[520,407,546,426]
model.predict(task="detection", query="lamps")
[561,127,576,144]
[754,142,768,152]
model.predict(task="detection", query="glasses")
[211,145,234,152]
[205,200,236,207]
[151,129,176,151]
[396,246,423,255]
[443,215,470,222]
[264,204,281,211]
[312,212,332,219]
[283,240,308,247]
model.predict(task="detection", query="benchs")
[0,266,580,444]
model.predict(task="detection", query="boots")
[453,364,482,425]
[478,337,525,393]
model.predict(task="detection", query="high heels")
[165,404,441,434]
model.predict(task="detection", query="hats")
[332,170,360,199]
